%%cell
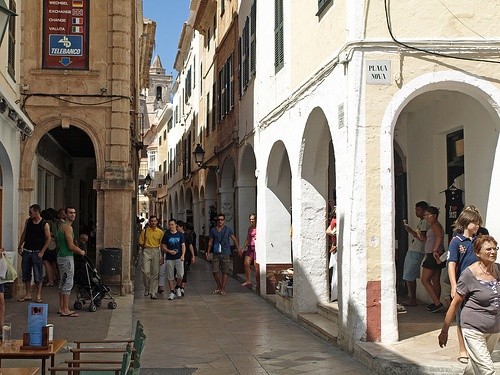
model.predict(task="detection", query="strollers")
[74,245,118,312]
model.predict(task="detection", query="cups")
[1,322,11,347]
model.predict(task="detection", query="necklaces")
[479,263,494,274]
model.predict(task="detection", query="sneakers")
[168,292,175,300]
[175,287,182,297]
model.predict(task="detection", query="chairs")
[48,320,147,375]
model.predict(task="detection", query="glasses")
[423,214,434,218]
[486,247,499,251]
[218,219,224,221]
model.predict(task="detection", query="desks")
[0,339,67,375]
[0,367,40,375]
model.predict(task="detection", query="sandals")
[42,282,55,287]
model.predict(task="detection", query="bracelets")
[433,250,439,252]
[441,330,448,334]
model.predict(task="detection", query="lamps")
[192,144,219,173]
[140,173,157,198]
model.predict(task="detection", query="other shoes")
[150,293,157,300]
[212,289,225,295]
[144,290,149,296]
[426,302,444,313]
[401,302,419,306]
[252,283,260,289]
[180,289,185,295]
[242,281,251,286]
[158,286,164,294]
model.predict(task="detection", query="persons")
[18,205,51,302]
[42,206,97,317]
[401,201,430,307]
[206,213,240,295]
[447,206,489,363]
[326,206,337,303]
[137,215,165,299]
[240,213,256,288]
[160,219,186,299]
[146,219,196,282]
[438,235,500,375]
[0,248,7,342]
[418,206,446,313]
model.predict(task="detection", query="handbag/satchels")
[4,255,17,280]
[214,243,221,254]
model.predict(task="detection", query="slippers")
[458,357,469,364]
[35,299,43,303]
[57,312,79,317]
[18,298,32,302]
